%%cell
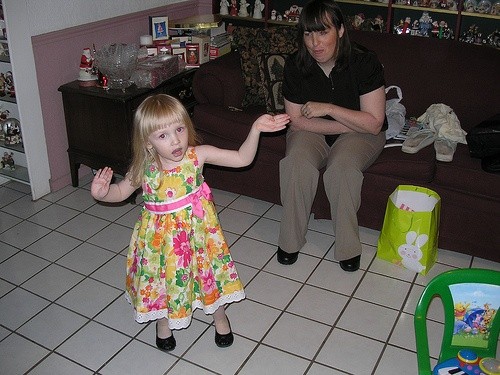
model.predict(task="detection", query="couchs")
[188,31,500,263]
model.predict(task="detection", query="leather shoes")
[339,255,360,272]
[276,246,300,265]
[214,315,234,348]
[155,322,176,352]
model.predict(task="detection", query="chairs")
[413,267,500,375]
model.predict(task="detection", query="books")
[166,12,233,61]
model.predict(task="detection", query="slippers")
[434,137,456,162]
[402,128,435,154]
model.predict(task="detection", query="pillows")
[258,52,290,116]
[231,24,309,111]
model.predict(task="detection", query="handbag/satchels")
[384,85,407,140]
[376,184,440,277]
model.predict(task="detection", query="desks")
[58,34,239,187]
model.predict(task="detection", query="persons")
[277,0,389,272]
[90,93,292,352]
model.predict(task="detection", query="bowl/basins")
[97,59,138,89]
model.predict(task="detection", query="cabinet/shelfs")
[212,0,499,50]
[0,1,31,187]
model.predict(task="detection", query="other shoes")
[466,131,500,175]
